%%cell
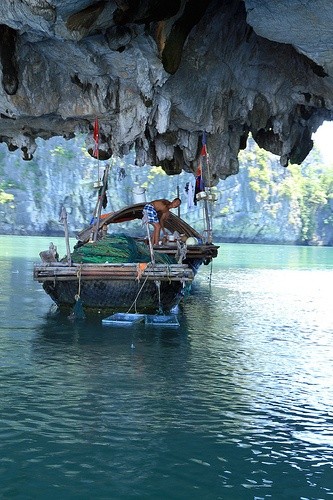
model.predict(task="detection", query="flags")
[193,158,205,206]
[98,170,108,209]
[93,117,99,157]
[200,128,207,157]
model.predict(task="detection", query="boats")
[34,206,195,316]
[74,116,219,277]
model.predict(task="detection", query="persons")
[143,198,182,246]
